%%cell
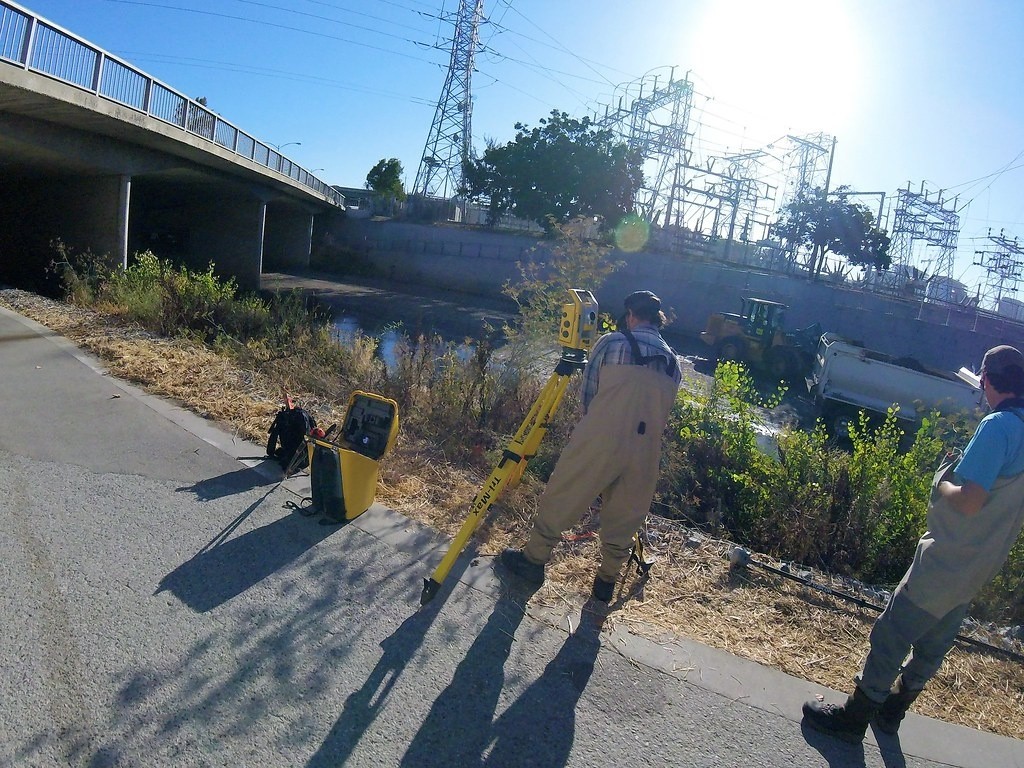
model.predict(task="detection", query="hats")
[975,345,1024,376]
[616,290,662,320]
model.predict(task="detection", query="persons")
[802,344,1024,745]
[502,289,681,600]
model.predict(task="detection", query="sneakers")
[593,575,616,601]
[502,548,544,584]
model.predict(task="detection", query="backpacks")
[264,406,317,468]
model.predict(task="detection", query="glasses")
[980,372,992,391]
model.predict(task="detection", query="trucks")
[811,331,992,441]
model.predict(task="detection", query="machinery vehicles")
[703,285,864,388]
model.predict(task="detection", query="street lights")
[265,140,303,169]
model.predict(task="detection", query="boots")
[802,686,884,746]
[873,673,923,736]
